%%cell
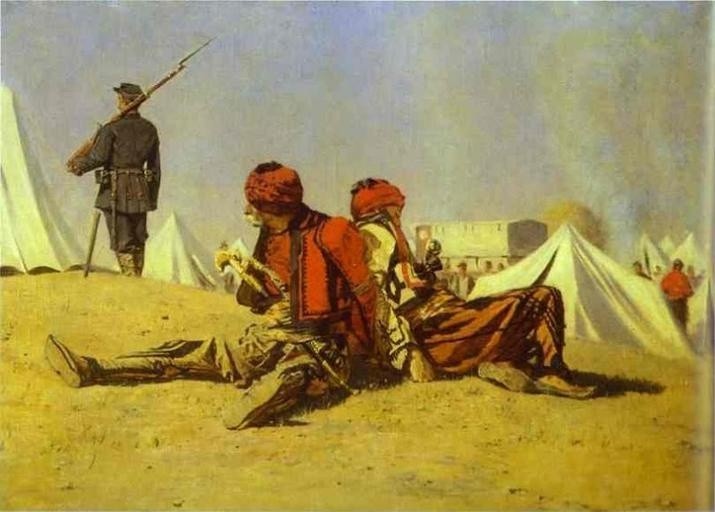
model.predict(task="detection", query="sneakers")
[533,372,601,399]
[221,375,286,428]
[478,360,536,393]
[45,333,91,386]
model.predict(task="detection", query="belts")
[107,167,144,175]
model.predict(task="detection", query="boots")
[114,247,144,277]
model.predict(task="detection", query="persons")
[343,175,600,400]
[65,83,162,277]
[445,262,476,303]
[43,160,379,433]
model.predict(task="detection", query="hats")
[112,83,145,100]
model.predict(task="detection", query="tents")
[454,220,698,361]
[0,81,94,276]
[100,210,229,294]
[622,222,715,363]
[218,235,257,290]
